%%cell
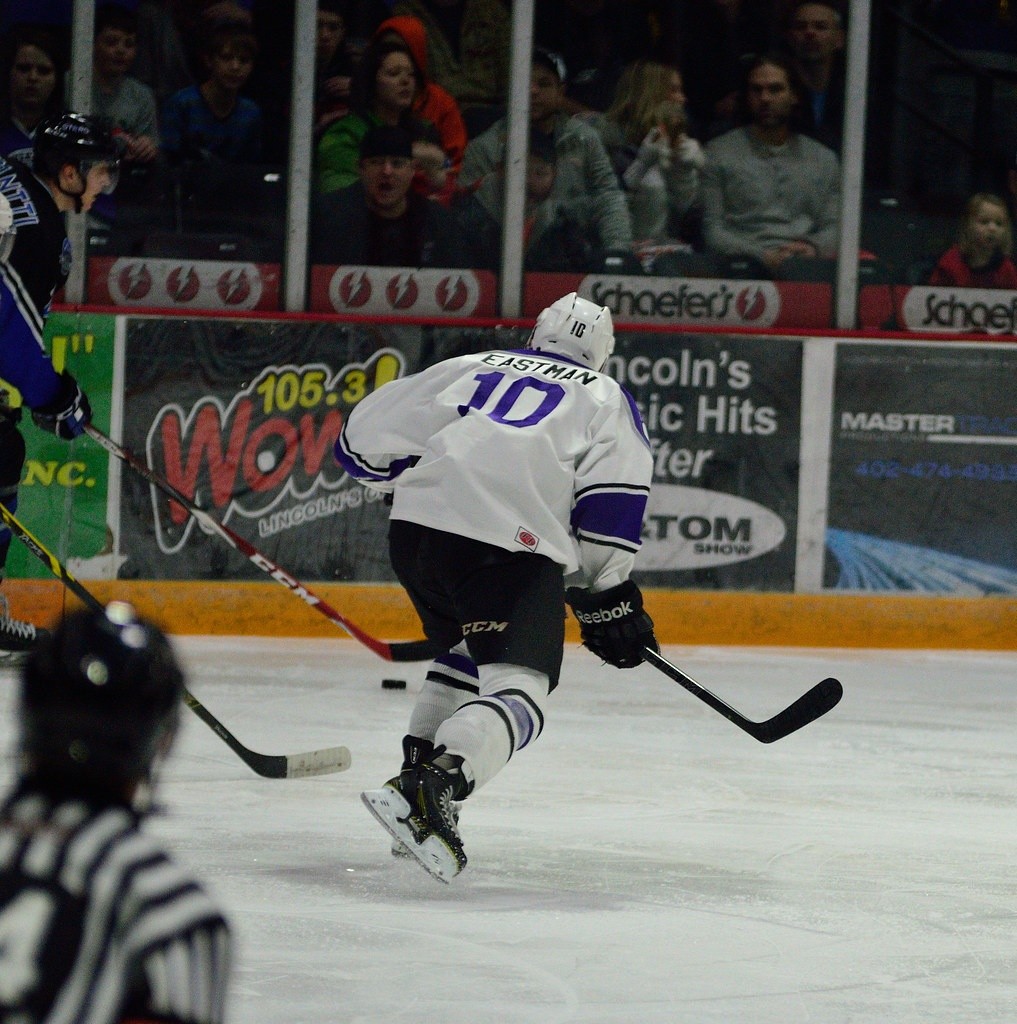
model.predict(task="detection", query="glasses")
[377,43,408,56]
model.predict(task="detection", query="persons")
[336,290,661,883]
[0,115,126,671]
[1,600,233,1024]
[1,0,1017,290]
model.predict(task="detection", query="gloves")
[571,581,658,669]
[32,372,93,442]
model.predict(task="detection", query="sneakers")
[361,744,468,884]
[389,735,433,858]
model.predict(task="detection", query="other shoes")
[0,616,50,670]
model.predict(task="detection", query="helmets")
[25,608,178,734]
[526,292,615,372]
[32,112,120,162]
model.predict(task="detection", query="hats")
[361,124,412,158]
[374,16,427,71]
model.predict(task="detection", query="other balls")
[382,679,407,689]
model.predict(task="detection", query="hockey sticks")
[80,420,434,662]
[0,502,352,780]
[381,491,844,744]
